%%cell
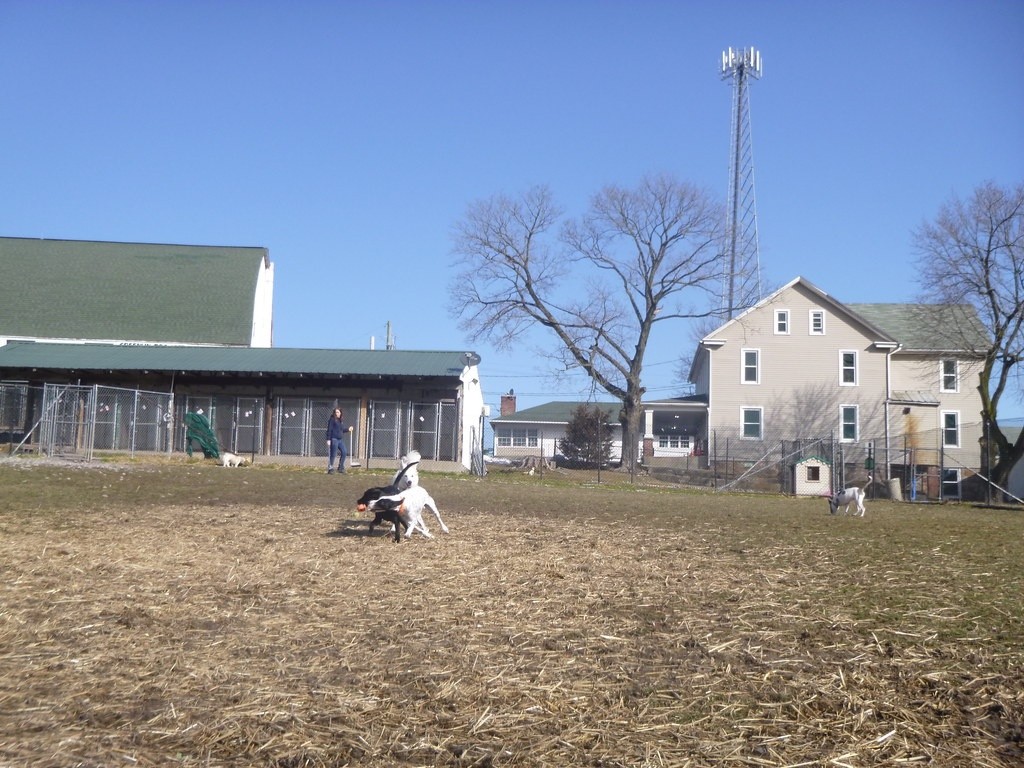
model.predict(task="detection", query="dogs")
[357,450,449,544]
[828,476,872,517]
[223,452,245,467]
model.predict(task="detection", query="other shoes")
[338,467,344,473]
[328,469,335,474]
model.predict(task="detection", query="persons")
[327,407,353,475]
